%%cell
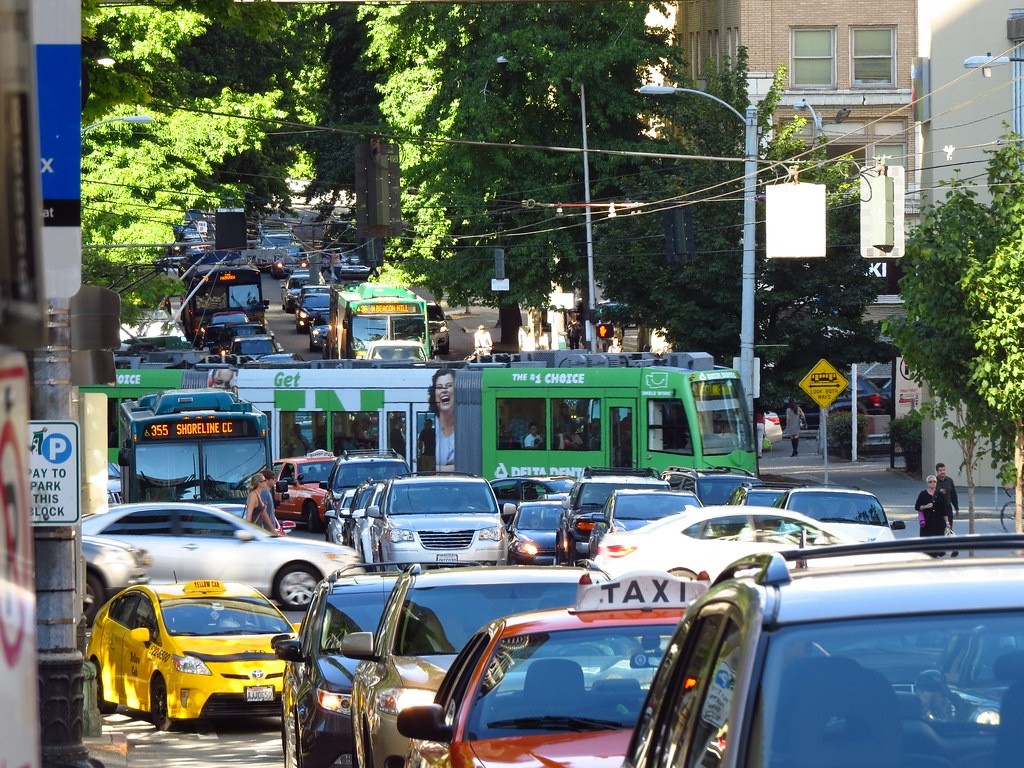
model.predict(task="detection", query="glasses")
[259,480,266,483]
[270,477,274,480]
[928,481,936,483]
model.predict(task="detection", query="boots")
[791,438,798,457]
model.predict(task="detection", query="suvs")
[775,371,891,431]
[621,530,1024,768]
[338,561,616,768]
[268,558,486,767]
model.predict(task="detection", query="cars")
[591,502,933,579]
[270,446,909,571]
[106,460,122,504]
[77,532,155,630]
[84,578,301,733]
[79,497,368,611]
[878,378,892,415]
[394,567,716,768]
[153,206,452,363]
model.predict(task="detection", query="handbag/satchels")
[274,525,285,537]
[943,526,955,537]
[918,511,927,528]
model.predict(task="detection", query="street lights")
[496,54,599,355]
[793,100,826,459]
[638,81,761,453]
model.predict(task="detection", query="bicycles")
[996,486,1024,533]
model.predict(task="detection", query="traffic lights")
[595,323,615,339]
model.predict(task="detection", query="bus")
[80,250,761,500]
[117,385,276,526]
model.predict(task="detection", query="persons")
[528,517,546,529]
[607,337,623,353]
[292,466,303,481]
[428,370,455,476]
[284,412,436,463]
[208,368,234,390]
[509,403,601,450]
[245,470,278,535]
[783,401,806,457]
[474,324,493,355]
[915,463,960,558]
[568,313,582,350]
[757,410,765,459]
[321,248,332,275]
